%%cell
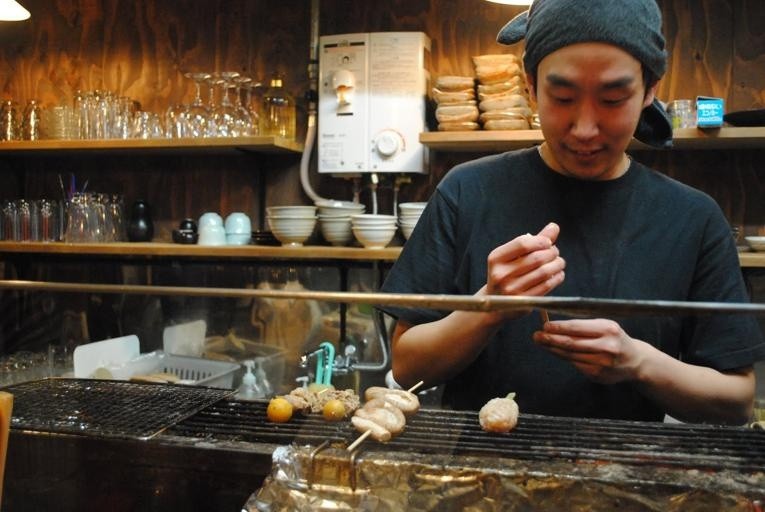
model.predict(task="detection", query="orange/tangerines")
[267,397,293,423]
[324,400,345,421]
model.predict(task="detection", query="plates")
[432,54,541,130]
[251,230,325,245]
[723,108,765,127]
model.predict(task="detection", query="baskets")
[107,353,241,389]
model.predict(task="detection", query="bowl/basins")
[265,200,428,249]
[173,211,251,246]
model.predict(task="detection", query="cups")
[0,90,165,142]
[671,99,696,128]
[0,191,124,244]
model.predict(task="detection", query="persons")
[373,0,765,425]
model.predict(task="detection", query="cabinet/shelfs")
[3,123,765,269]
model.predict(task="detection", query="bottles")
[260,69,296,142]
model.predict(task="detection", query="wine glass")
[166,72,261,137]
